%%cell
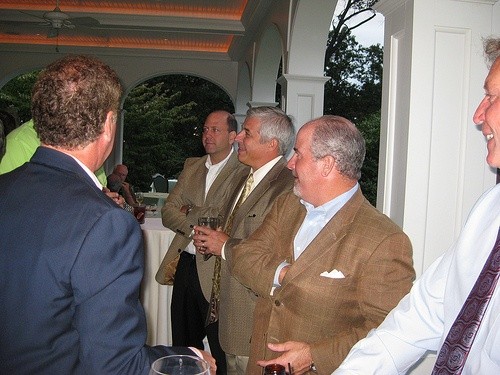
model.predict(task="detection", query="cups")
[133,206,146,224]
[149,355,210,375]
[197,217,221,235]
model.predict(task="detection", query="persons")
[193,105,297,375]
[332,39,500,375]
[232,114,416,375]
[0,53,217,375]
[156,111,251,375]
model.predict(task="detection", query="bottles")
[264,363,285,375]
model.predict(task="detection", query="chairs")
[152,174,168,193]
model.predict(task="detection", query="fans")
[12,0,99,52]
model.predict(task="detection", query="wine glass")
[135,191,144,206]
[151,206,156,216]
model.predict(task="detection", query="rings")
[200,247,203,250]
[203,243,205,247]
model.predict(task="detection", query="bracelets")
[309,361,317,372]
[186,205,192,216]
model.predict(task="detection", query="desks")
[140,218,176,347]
[135,180,179,217]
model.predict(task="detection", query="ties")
[209,172,254,304]
[431,227,500,375]
